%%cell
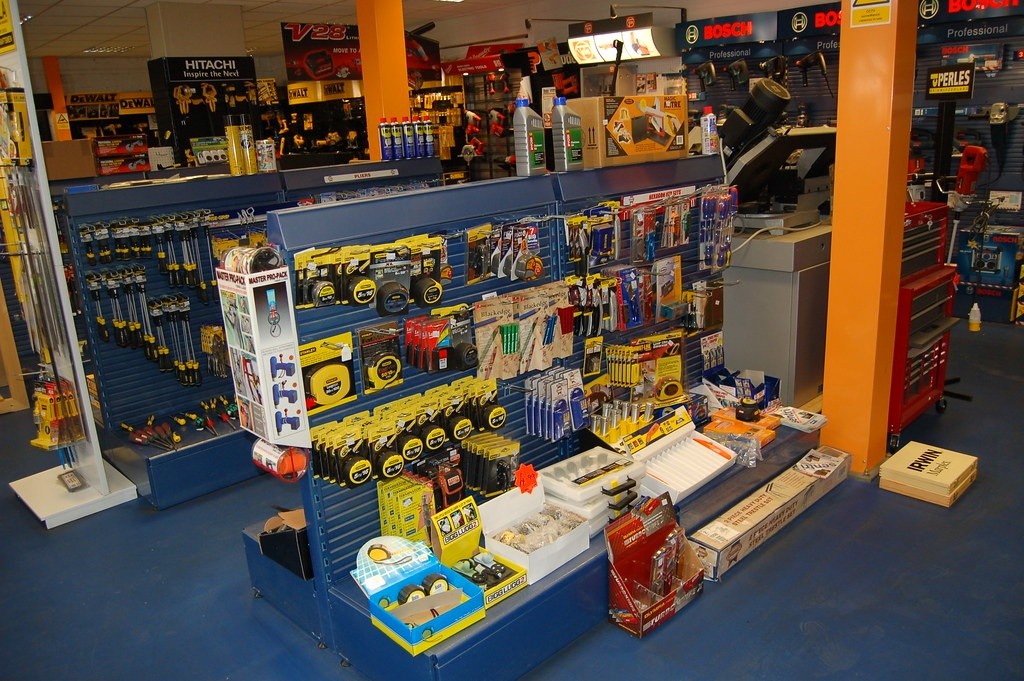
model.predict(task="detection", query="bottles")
[699,106,720,156]
[512,97,547,177]
[377,118,394,162]
[422,115,435,158]
[551,96,585,172]
[911,159,926,202]
[389,116,406,161]
[412,115,426,159]
[401,116,417,160]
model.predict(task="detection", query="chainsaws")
[462,138,484,162]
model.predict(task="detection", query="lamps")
[525,18,594,29]
[610,3,688,24]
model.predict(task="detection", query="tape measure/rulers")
[368,544,392,561]
[398,573,449,607]
[309,275,506,489]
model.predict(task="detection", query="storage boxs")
[586,490,642,539]
[685,444,852,583]
[879,441,979,496]
[707,368,780,410]
[711,407,782,431]
[879,470,977,508]
[349,534,486,659]
[94,153,151,176]
[652,392,709,425]
[952,282,1020,323]
[256,508,315,582]
[603,491,705,641]
[214,264,313,451]
[704,419,776,449]
[533,445,647,508]
[623,405,737,505]
[40,139,101,182]
[956,224,1024,290]
[565,95,689,168]
[92,133,148,157]
[544,478,641,523]
[478,478,589,587]
[147,56,267,167]
[430,495,528,610]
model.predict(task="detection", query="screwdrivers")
[703,186,739,267]
[78,210,221,387]
[121,393,238,451]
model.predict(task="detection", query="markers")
[500,324,519,354]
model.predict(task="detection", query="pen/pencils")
[474,296,560,381]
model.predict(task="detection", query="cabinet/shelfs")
[0,0,140,531]
[62,169,286,513]
[242,152,817,681]
